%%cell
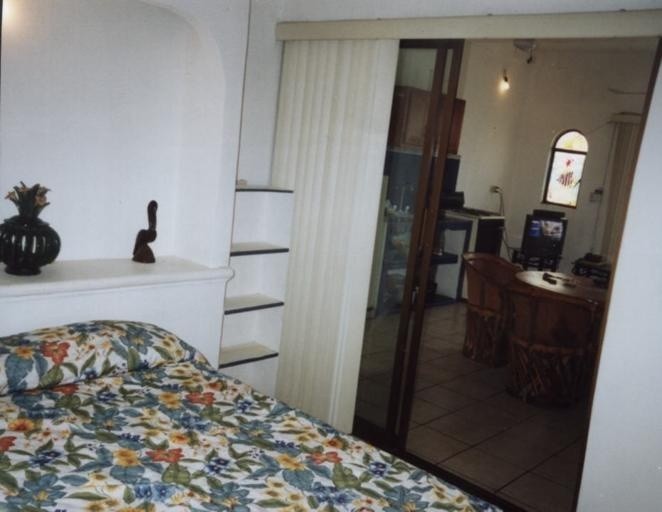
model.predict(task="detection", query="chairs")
[463,252,522,368]
[506,284,598,411]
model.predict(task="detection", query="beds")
[0,269,508,512]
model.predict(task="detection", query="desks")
[516,270,606,348]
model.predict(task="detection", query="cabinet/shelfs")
[377,215,473,315]
[217,186,291,368]
[511,246,563,271]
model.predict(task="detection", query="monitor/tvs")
[520,213,568,255]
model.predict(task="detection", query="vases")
[0,217,60,275]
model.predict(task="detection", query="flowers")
[5,181,51,218]
